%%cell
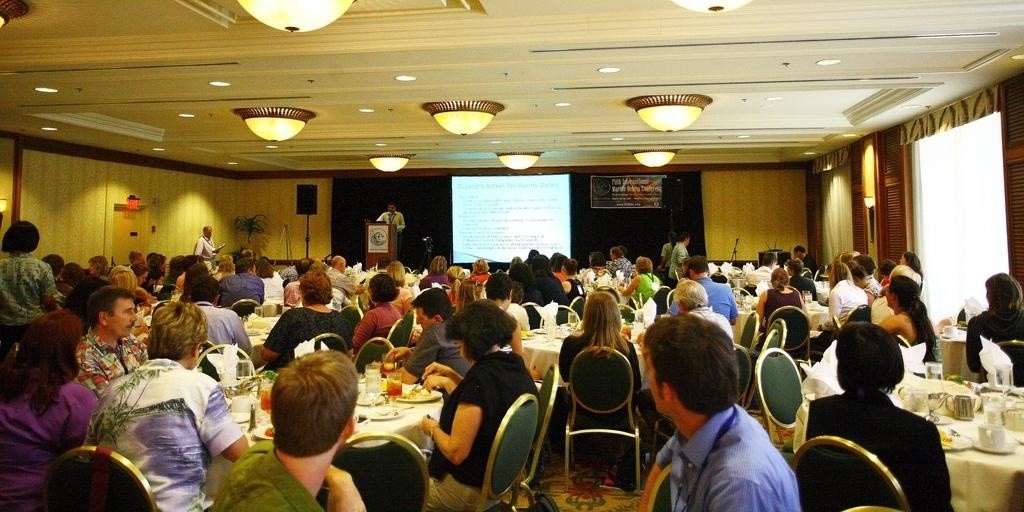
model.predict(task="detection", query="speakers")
[297,185,317,215]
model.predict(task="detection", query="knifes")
[411,377,426,392]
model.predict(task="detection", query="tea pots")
[945,394,976,420]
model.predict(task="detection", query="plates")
[972,440,1018,454]
[357,410,406,421]
[357,394,386,407]
[395,389,443,404]
[940,334,961,339]
[532,330,546,334]
[251,425,274,441]
[941,436,973,452]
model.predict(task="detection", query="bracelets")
[428,426,438,441]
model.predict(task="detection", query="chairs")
[43,445,158,511]
[651,286,673,315]
[542,304,580,325]
[766,305,812,367]
[569,294,586,321]
[194,343,256,380]
[617,304,636,324]
[522,305,541,330]
[386,318,409,346]
[813,269,821,281]
[314,332,349,354]
[510,362,560,512]
[801,268,813,279]
[761,329,779,351]
[736,311,761,352]
[440,284,451,290]
[315,431,429,512]
[709,272,728,284]
[628,296,638,311]
[790,435,910,512]
[666,288,676,309]
[739,283,758,297]
[736,328,779,411]
[230,297,260,316]
[595,285,621,304]
[754,347,805,447]
[353,337,394,374]
[732,342,751,403]
[565,345,642,495]
[766,318,788,349]
[203,341,220,354]
[403,309,417,347]
[980,340,1024,388]
[638,292,647,309]
[475,392,539,511]
[729,286,751,297]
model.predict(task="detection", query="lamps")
[419,100,505,136]
[863,197,875,208]
[0,198,8,213]
[625,94,713,133]
[368,153,416,172]
[233,106,316,143]
[237,0,356,33]
[496,152,542,170]
[0,0,29,28]
[669,0,755,15]
[630,149,681,168]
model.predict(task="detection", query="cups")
[260,385,274,437]
[255,306,263,317]
[982,398,1005,427]
[625,278,632,287]
[365,377,382,410]
[802,290,810,302]
[745,295,754,312]
[386,373,403,407]
[924,361,944,380]
[232,395,252,424]
[978,425,1005,451]
[568,312,578,325]
[943,326,957,337]
[365,364,381,377]
[736,293,744,305]
[236,360,251,380]
[804,292,813,303]
[635,309,645,323]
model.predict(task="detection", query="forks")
[376,406,415,417]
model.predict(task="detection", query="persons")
[418,255,451,291]
[634,313,803,512]
[325,253,365,311]
[531,254,569,307]
[175,255,203,294]
[0,308,96,512]
[128,249,145,266]
[559,291,642,492]
[667,254,737,326]
[447,265,467,310]
[672,278,736,351]
[351,273,404,354]
[560,258,586,304]
[869,263,922,325]
[141,252,168,294]
[362,256,393,312]
[71,286,148,398]
[41,253,86,295]
[163,254,185,287]
[212,254,236,282]
[606,245,632,282]
[785,257,818,302]
[181,263,209,299]
[508,256,523,276]
[964,271,1024,389]
[794,318,955,512]
[372,201,406,258]
[255,255,285,305]
[619,255,662,308]
[836,247,883,308]
[665,231,692,291]
[793,244,819,281]
[88,255,110,281]
[194,224,218,271]
[549,252,569,282]
[0,219,59,338]
[898,250,921,274]
[259,270,354,372]
[746,251,782,290]
[217,260,265,316]
[656,231,678,278]
[875,258,896,287]
[130,264,149,287]
[876,274,936,378]
[282,257,316,307]
[190,274,251,353]
[238,248,255,260]
[523,248,541,265]
[465,258,491,284]
[72,298,250,512]
[508,262,543,305]
[578,250,614,293]
[383,287,472,385]
[209,348,369,512]
[484,271,542,381]
[420,299,541,512]
[455,278,482,315]
[811,260,868,353]
[155,283,177,302]
[755,267,811,328]
[386,258,413,316]
[107,264,138,294]
